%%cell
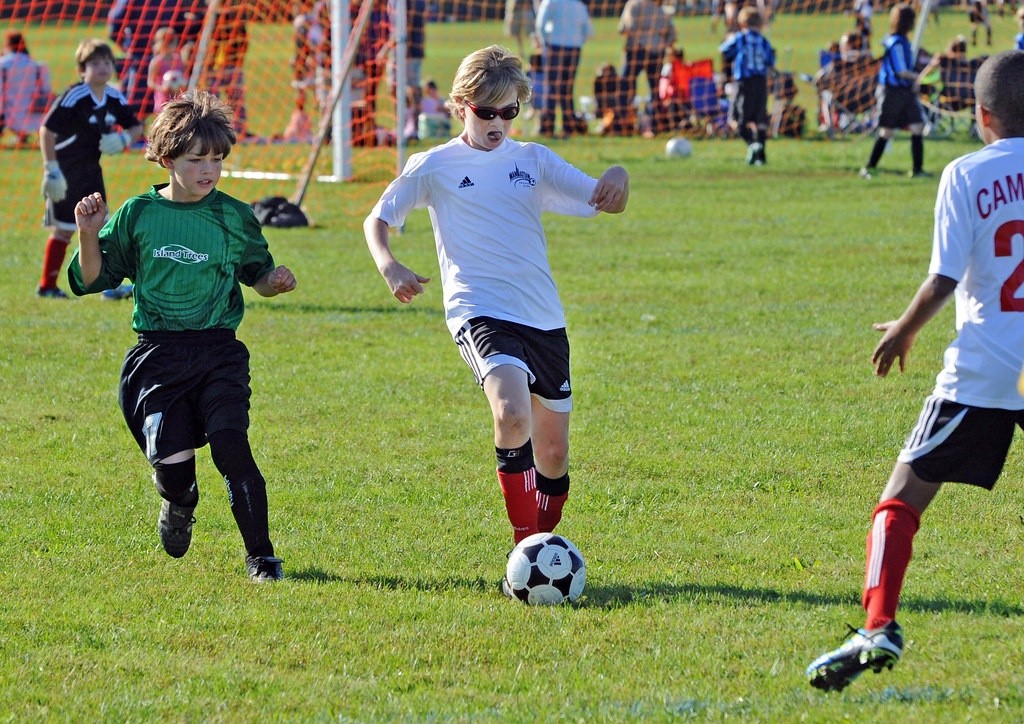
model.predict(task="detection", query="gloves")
[99,130,133,155]
[41,161,69,203]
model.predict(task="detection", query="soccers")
[506,532,587,606]
[666,136,693,159]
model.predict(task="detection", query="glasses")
[464,95,522,120]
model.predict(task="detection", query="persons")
[67,86,297,584]
[0,31,49,146]
[815,0,1024,180]
[807,50,1024,692]
[709,0,777,167]
[421,78,451,118]
[362,42,631,548]
[284,0,425,145]
[505,0,590,138]
[616,0,676,113]
[594,64,632,137]
[35,38,142,300]
[106,0,247,128]
[665,46,690,130]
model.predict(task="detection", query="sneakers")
[157,499,197,559]
[103,283,134,299]
[910,169,935,180]
[860,167,879,179]
[745,141,764,167]
[807,618,904,691]
[243,549,287,585]
[34,283,70,298]
